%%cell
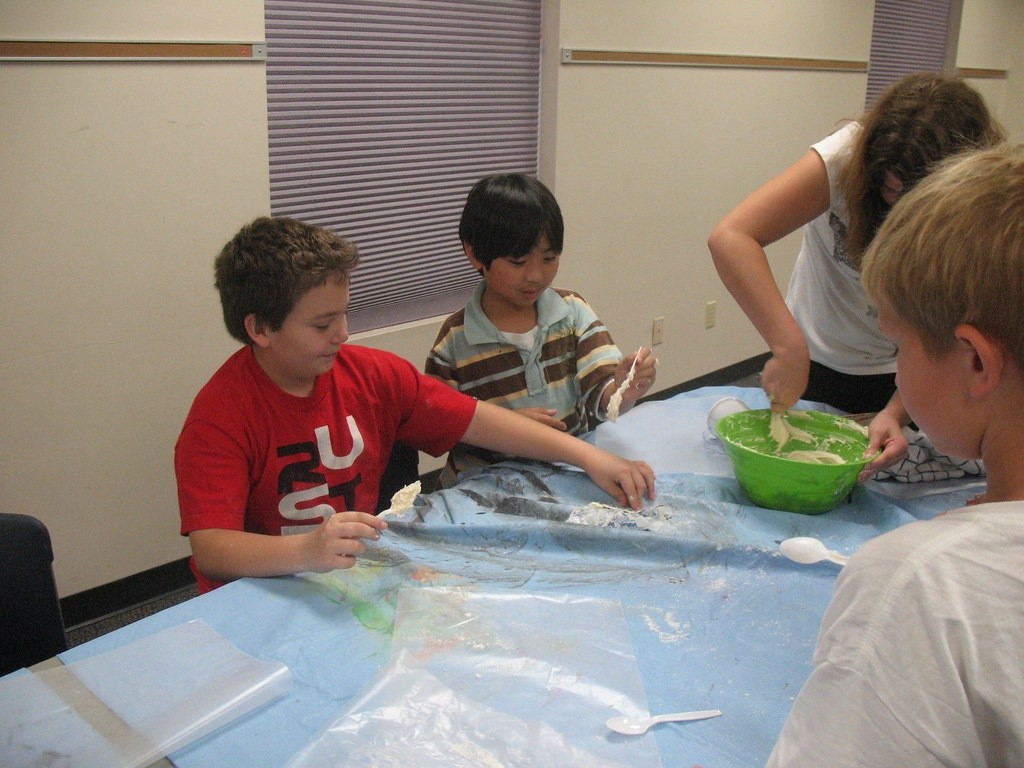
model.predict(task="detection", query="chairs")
[0,511,66,677]
[374,443,419,515]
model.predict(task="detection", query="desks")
[0,384,987,768]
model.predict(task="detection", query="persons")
[424,173,656,495]
[767,142,1024,768]
[174,218,656,596]
[707,72,1009,486]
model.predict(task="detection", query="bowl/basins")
[716,405,882,513]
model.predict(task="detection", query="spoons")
[778,536,849,566]
[376,481,421,517]
[605,708,722,735]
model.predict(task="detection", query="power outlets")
[705,301,718,329]
[652,316,663,346]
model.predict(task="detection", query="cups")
[706,397,752,438]
